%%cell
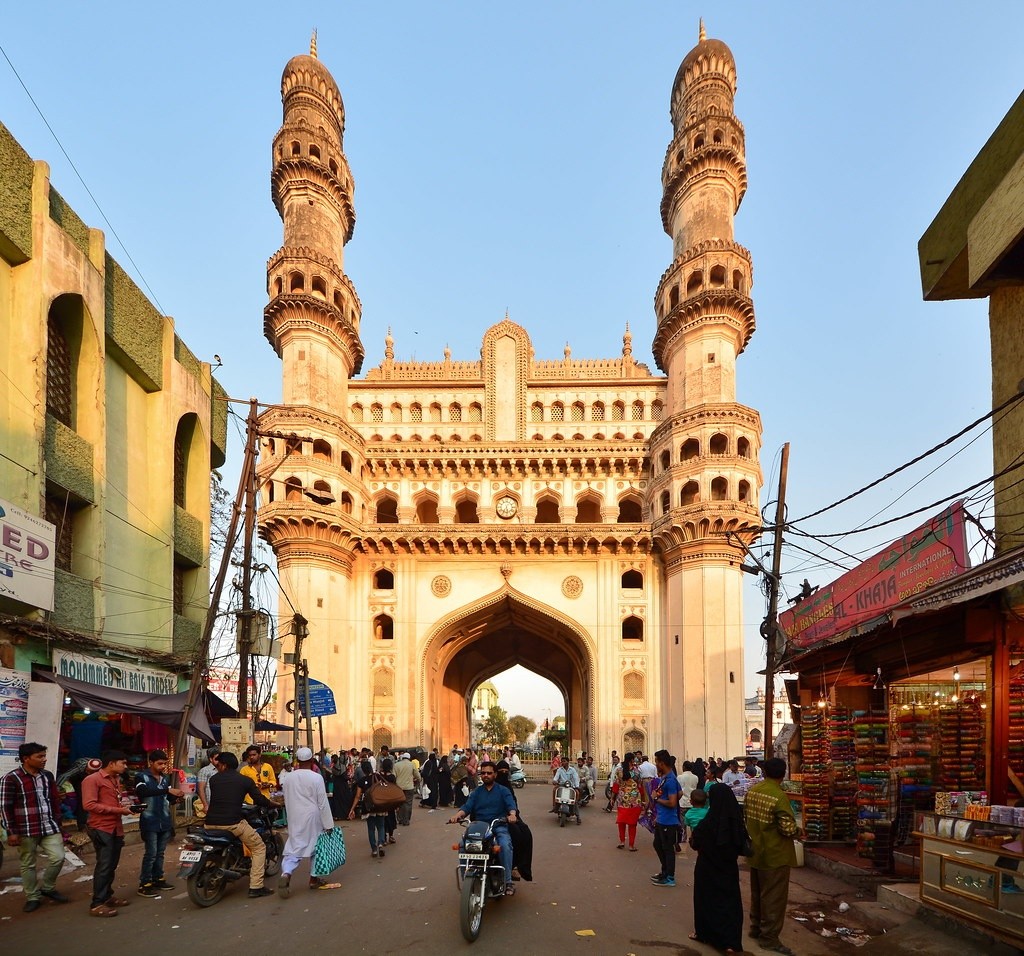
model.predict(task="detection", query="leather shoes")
[248,886,275,898]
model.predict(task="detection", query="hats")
[297,747,312,761]
[457,748,463,752]
[402,752,411,760]
[496,760,510,770]
[87,759,103,770]
[215,752,238,769]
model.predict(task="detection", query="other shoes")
[384,836,396,846]
[617,845,639,851]
[505,884,516,896]
[23,900,40,912]
[372,845,385,857]
[40,887,68,902]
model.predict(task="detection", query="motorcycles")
[174,803,286,908]
[444,816,522,943]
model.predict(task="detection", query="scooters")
[577,778,597,809]
[508,767,527,789]
[605,782,612,798]
[549,779,578,827]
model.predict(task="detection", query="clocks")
[497,497,517,517]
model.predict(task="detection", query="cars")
[529,747,541,756]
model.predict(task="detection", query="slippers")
[104,897,129,906]
[89,905,118,917]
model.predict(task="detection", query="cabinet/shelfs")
[800,647,1024,950]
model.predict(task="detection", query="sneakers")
[136,883,159,897]
[152,876,176,890]
[650,873,675,886]
[279,873,290,898]
[310,878,329,889]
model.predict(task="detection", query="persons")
[239,746,277,804]
[651,749,683,887]
[278,745,521,821]
[377,759,399,846]
[204,751,281,898]
[0,742,69,912]
[278,747,334,899]
[449,760,533,895]
[683,788,708,830]
[392,753,423,826]
[745,758,800,953]
[348,761,387,858]
[196,748,222,815]
[683,782,750,955]
[987,799,1023,895]
[236,751,250,772]
[56,756,102,831]
[134,750,180,897]
[80,750,137,917]
[545,743,762,852]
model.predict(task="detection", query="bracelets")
[799,677,1024,862]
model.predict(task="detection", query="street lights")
[541,707,552,724]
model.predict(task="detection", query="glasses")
[480,771,494,776]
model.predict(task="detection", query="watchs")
[654,796,658,802]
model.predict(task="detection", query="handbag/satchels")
[310,826,346,876]
[364,773,406,812]
